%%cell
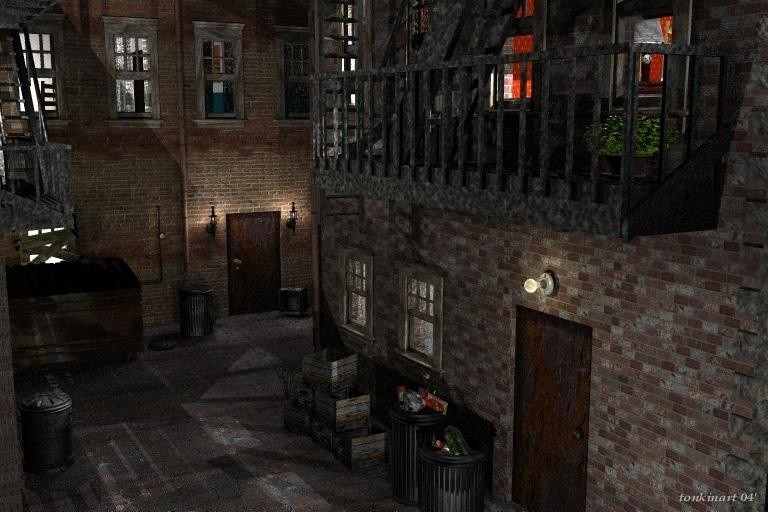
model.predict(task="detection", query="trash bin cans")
[18,390,76,475]
[178,284,214,337]
[388,401,488,512]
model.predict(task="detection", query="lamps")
[206,205,218,242]
[286,201,298,232]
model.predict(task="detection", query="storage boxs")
[283,348,391,470]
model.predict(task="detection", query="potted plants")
[581,112,679,178]
